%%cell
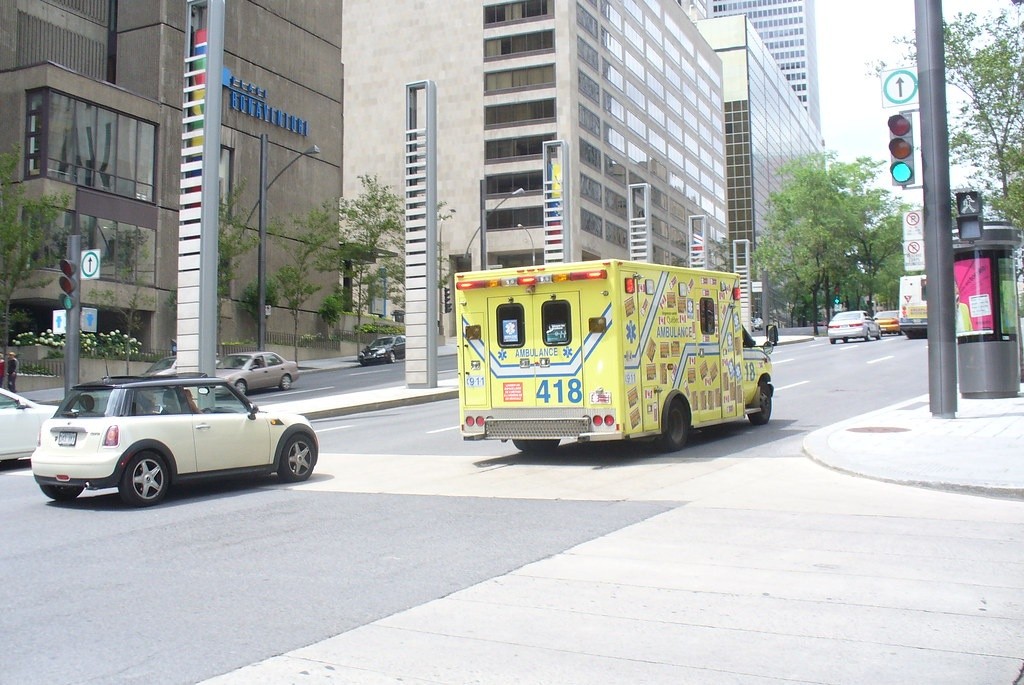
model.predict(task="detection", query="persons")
[8,352,19,393]
[0,352,5,387]
[185,390,203,414]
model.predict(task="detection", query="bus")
[897,273,928,340]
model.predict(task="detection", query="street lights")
[217,145,320,362]
[464,187,525,271]
[517,223,537,266]
[438,207,458,337]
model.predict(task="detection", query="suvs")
[28,371,320,509]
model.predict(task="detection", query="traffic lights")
[444,287,453,313]
[57,258,78,310]
[887,113,916,186]
[833,282,840,305]
[954,190,984,241]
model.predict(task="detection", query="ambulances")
[452,257,780,456]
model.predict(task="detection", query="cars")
[749,315,763,332]
[216,351,301,400]
[825,309,882,344]
[0,387,81,459]
[357,335,405,366]
[872,310,902,336]
[142,356,178,390]
